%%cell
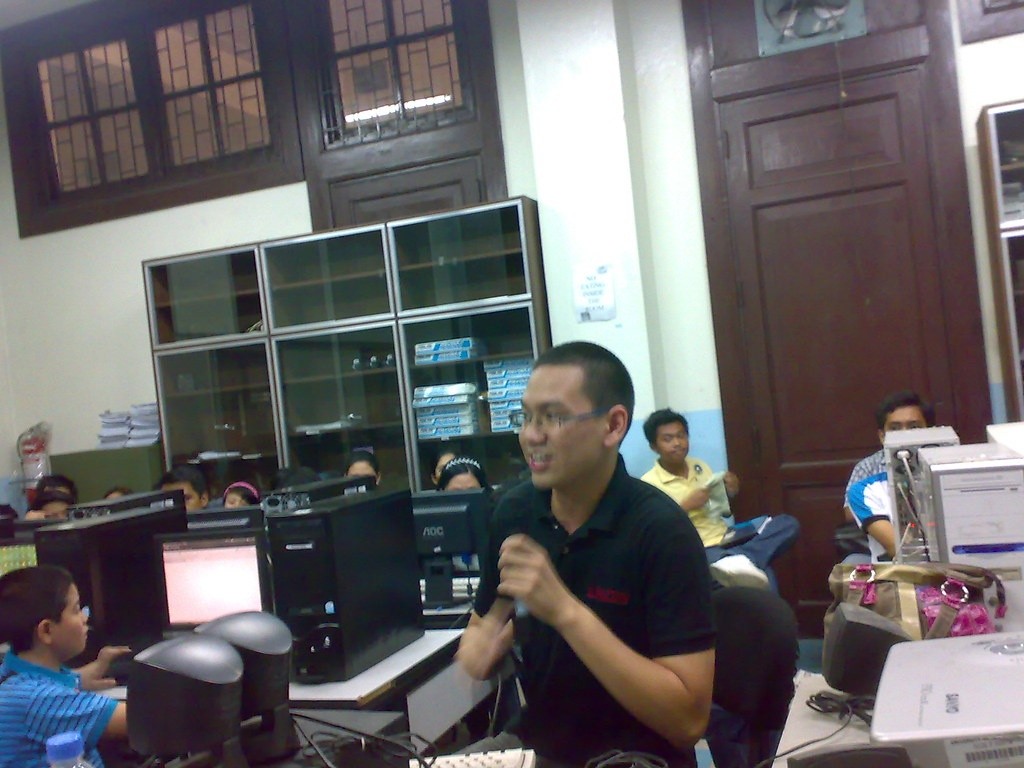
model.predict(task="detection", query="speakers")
[821,602,914,697]
[125,611,304,768]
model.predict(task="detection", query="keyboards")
[405,750,535,767]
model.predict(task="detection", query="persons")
[637,407,803,605]
[0,564,133,768]
[844,389,939,558]
[457,340,716,768]
[0,449,494,521]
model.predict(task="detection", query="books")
[718,511,772,546]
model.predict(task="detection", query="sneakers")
[710,554,768,589]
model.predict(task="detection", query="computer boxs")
[884,425,961,565]
[261,476,426,687]
[32,489,188,687]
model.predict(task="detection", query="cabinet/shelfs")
[142,196,553,502]
[976,99,1024,424]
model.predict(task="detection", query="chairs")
[695,586,800,768]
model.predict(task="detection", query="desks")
[97,576,520,768]
[52,445,166,505]
[773,674,875,768]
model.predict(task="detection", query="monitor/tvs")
[918,421,1023,581]
[0,518,71,577]
[412,486,489,608]
[152,505,273,642]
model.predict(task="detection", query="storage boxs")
[484,357,534,432]
[414,337,487,366]
[413,382,479,440]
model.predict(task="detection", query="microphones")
[493,495,533,650]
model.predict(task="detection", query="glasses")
[508,407,611,433]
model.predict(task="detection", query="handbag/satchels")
[821,562,1006,659]
